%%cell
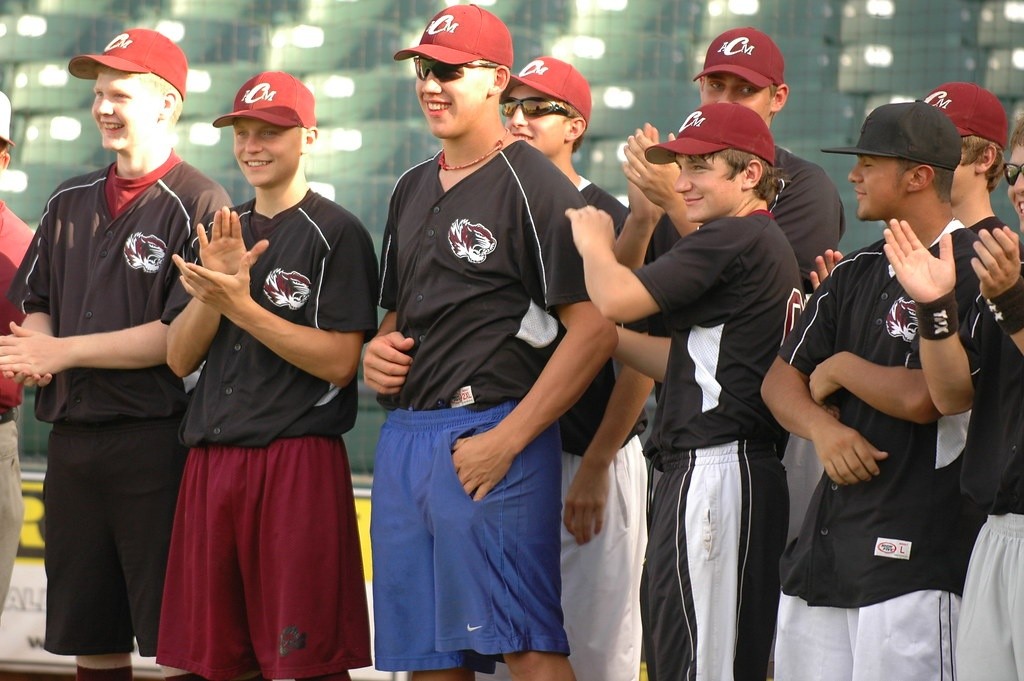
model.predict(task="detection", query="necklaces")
[436,128,510,172]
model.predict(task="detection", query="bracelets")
[899,290,960,343]
[981,279,1024,334]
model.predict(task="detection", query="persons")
[0,91,34,625]
[499,26,1024,681]
[362,3,619,680]
[162,68,378,681]
[1,28,244,681]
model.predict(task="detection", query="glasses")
[501,97,577,119]
[1003,163,1024,187]
[414,57,510,83]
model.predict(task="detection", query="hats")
[212,70,316,130]
[0,92,15,147]
[645,102,775,167]
[499,56,591,139]
[821,98,962,171]
[393,5,514,70]
[69,28,187,101]
[923,82,1007,149]
[693,26,784,89]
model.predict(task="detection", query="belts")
[0,412,15,424]
